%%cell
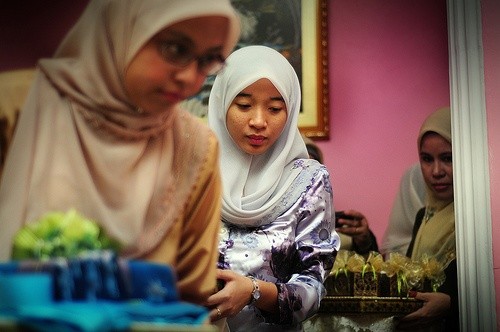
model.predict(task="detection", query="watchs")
[248,275,262,305]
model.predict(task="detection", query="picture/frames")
[176,0,331,141]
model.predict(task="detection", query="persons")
[0,0,243,330]
[302,134,381,257]
[389,106,462,330]
[200,44,341,331]
[377,162,432,259]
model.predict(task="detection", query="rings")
[216,307,221,318]
